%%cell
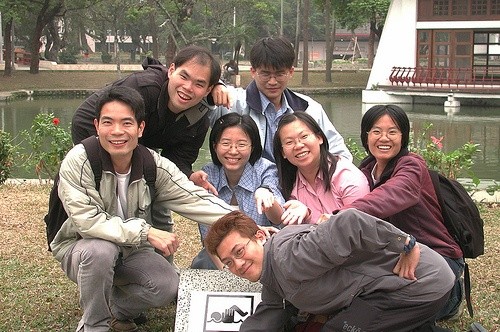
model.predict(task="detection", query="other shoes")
[466,322,488,332]
[167,262,182,276]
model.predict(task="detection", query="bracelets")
[253,184,274,196]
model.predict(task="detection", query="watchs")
[401,234,416,255]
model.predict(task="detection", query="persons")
[204,207,491,332]
[204,36,354,164]
[72,46,221,273]
[50,85,281,332]
[316,104,472,321]
[273,111,371,225]
[190,112,286,270]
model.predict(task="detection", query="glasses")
[281,131,313,148]
[255,69,290,82]
[216,136,251,152]
[222,233,256,271]
[367,125,403,141]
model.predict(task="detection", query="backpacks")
[430,168,485,259]
[44,136,157,251]
